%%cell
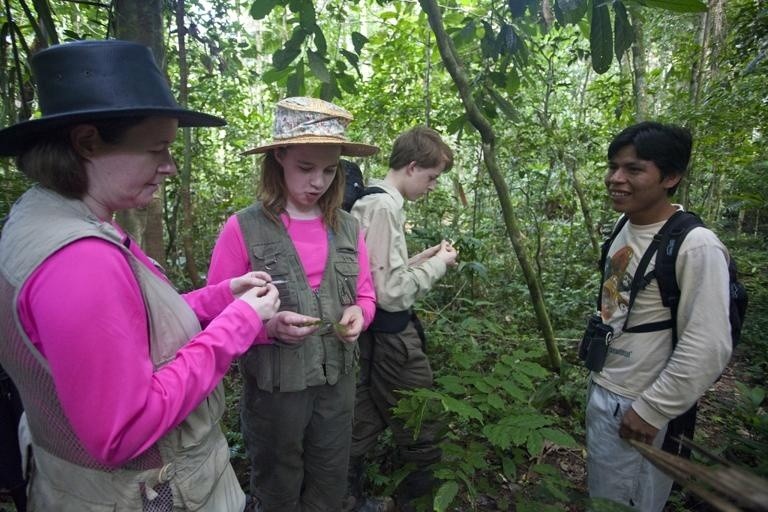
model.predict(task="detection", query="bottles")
[240,97,381,157]
[1,41,227,154]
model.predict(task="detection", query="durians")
[580,315,613,372]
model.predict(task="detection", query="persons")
[583,123,734,512]
[204,96,376,512]
[346,126,459,511]
[1,40,281,512]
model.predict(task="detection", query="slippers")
[597,211,749,385]
[339,159,414,337]
